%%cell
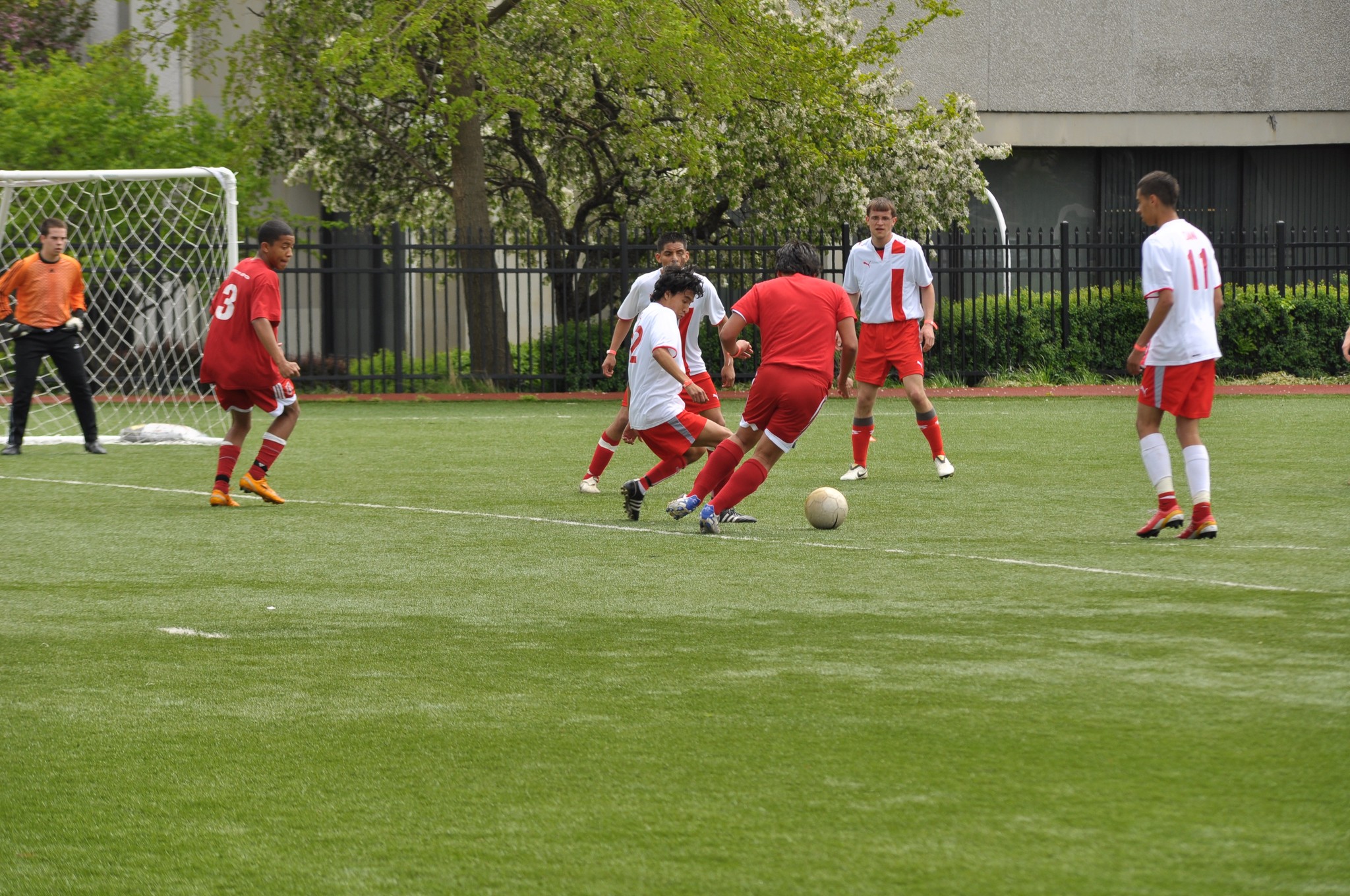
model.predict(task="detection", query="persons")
[579,232,756,524]
[834,197,954,479]
[667,242,857,534]
[1128,172,1224,541]
[1342,326,1350,361]
[0,217,107,455]
[201,221,301,506]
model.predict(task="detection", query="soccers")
[805,487,849,529]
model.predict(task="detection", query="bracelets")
[1133,343,1147,352]
[727,344,741,366]
[606,350,616,355]
[683,379,693,388]
[924,319,938,330]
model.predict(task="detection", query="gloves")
[66,317,84,333]
[9,323,33,338]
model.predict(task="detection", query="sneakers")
[579,475,600,493]
[1176,515,1218,540]
[210,488,240,507]
[665,492,701,521]
[621,478,645,522]
[934,455,955,480]
[839,463,867,481]
[240,471,286,505]
[1,444,20,455]
[719,507,758,523]
[699,503,721,535]
[85,441,107,454]
[1137,505,1184,538]
